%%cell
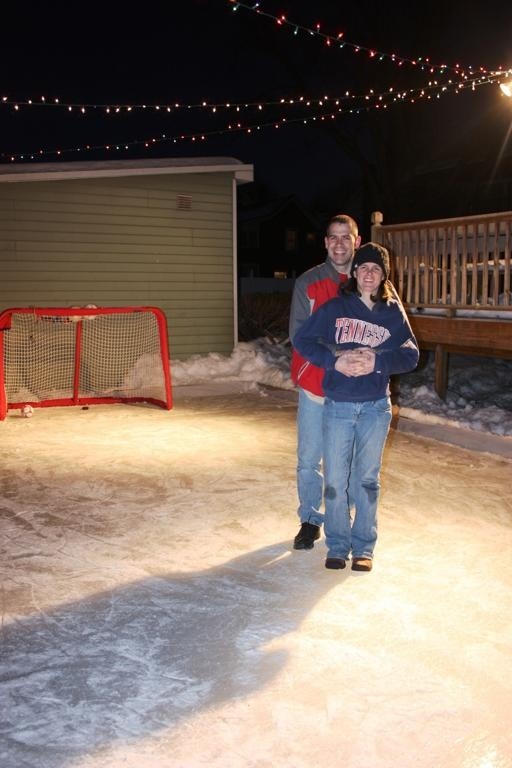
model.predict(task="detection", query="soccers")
[21,405,33,418]
[68,303,98,322]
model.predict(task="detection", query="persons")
[292,240,422,569]
[286,213,402,552]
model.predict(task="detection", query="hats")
[351,242,391,281]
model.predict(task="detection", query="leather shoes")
[325,558,346,569]
[351,558,372,571]
[294,522,320,550]
